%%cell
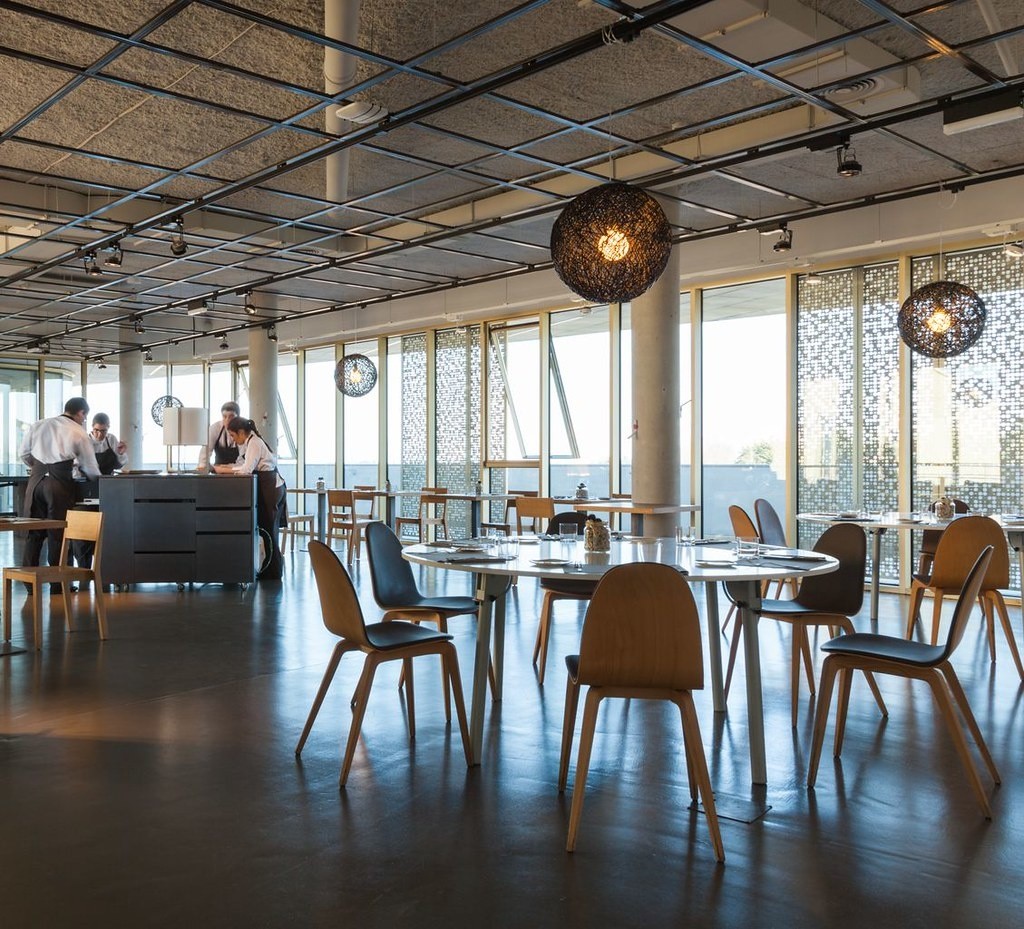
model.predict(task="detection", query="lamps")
[334,302,377,397]
[773,222,793,252]
[897,179,986,359]
[836,143,862,177]
[550,42,672,306]
[163,407,211,474]
[83,215,277,370]
[151,341,184,427]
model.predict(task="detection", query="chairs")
[2,510,109,652]
[278,485,1024,863]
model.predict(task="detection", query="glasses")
[94,429,108,433]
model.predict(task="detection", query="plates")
[698,562,736,566]
[896,520,922,523]
[1002,520,1024,525]
[530,560,570,565]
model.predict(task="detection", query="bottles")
[316,477,325,490]
[583,521,610,552]
[576,483,588,499]
[386,479,391,491]
[935,498,956,518]
[476,481,482,495]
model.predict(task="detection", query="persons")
[197,402,241,470]
[20,397,102,595]
[73,413,129,590]
[213,417,288,580]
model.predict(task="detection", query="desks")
[573,503,702,536]
[0,474,259,593]
[427,493,524,540]
[286,490,342,552]
[352,491,434,534]
[401,533,840,785]
[0,517,68,656]
[795,511,1024,621]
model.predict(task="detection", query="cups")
[675,526,696,546]
[737,537,759,560]
[478,527,521,559]
[559,523,578,541]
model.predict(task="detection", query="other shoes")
[51,586,78,593]
[102,585,111,593]
[26,587,33,595]
[257,571,282,579]
